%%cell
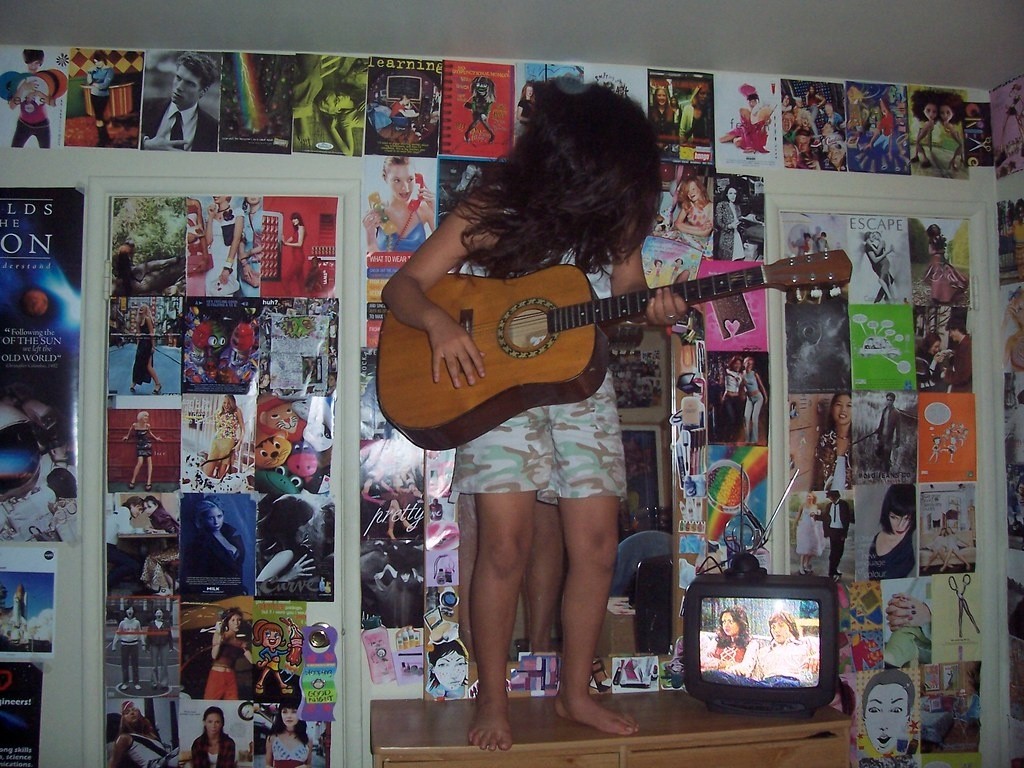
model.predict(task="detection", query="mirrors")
[458,425,676,664]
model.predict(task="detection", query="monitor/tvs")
[682,571,840,718]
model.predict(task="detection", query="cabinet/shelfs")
[368,692,854,768]
[788,393,817,490]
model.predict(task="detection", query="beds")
[918,695,959,750]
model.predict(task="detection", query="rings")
[666,314,676,319]
[304,569,306,572]
[367,216,373,221]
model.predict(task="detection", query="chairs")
[164,561,180,595]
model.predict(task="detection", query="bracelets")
[224,262,232,269]
[224,267,233,274]
[226,258,233,263]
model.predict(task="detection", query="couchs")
[920,531,977,567]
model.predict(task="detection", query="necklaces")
[250,211,256,214]
[839,436,848,440]
[218,205,230,212]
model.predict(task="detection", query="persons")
[700,606,761,678]
[10,48,967,180]
[702,610,820,688]
[605,164,1024,583]
[381,77,688,752]
[107,156,436,768]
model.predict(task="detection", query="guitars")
[374,245,854,453]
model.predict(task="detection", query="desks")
[118,533,178,567]
